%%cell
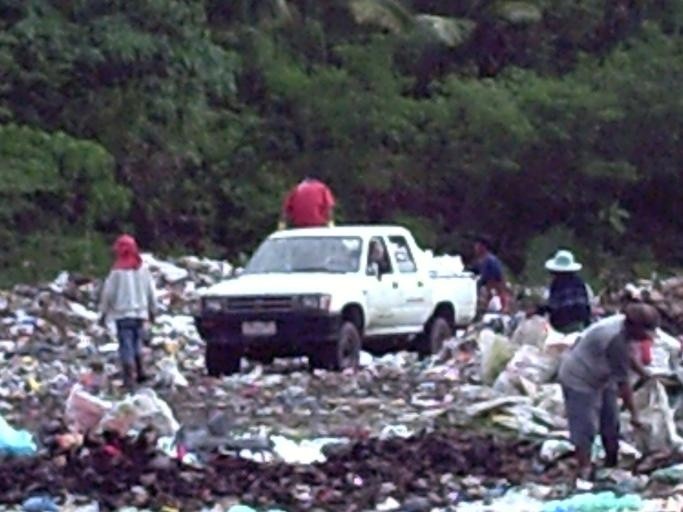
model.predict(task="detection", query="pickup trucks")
[192,224,478,376]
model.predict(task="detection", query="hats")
[544,248,582,273]
[471,227,502,255]
[621,302,664,340]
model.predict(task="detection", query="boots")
[118,355,156,389]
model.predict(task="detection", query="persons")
[529,250,591,333]
[96,235,157,393]
[560,303,658,469]
[368,240,387,272]
[277,168,337,230]
[468,238,513,314]
